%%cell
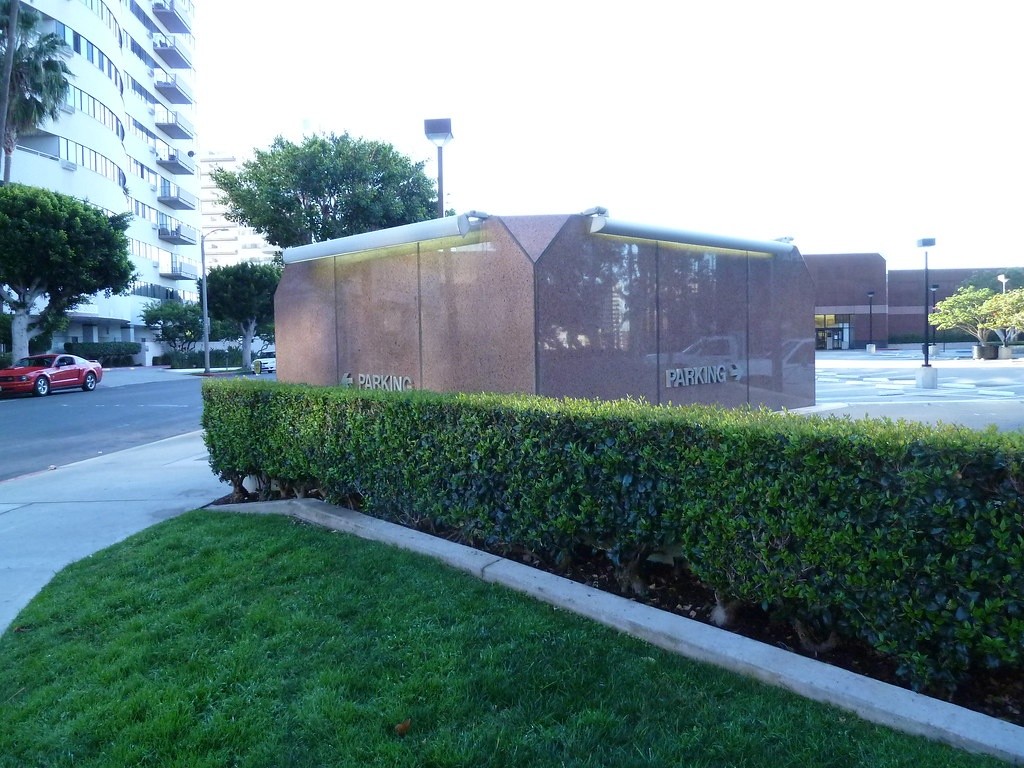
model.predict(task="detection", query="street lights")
[928,283,940,347]
[998,274,1012,294]
[425,118,454,220]
[917,237,936,368]
[200,227,229,373]
[867,291,875,345]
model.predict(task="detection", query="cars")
[251,350,276,374]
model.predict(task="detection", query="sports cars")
[0,353,103,398]
